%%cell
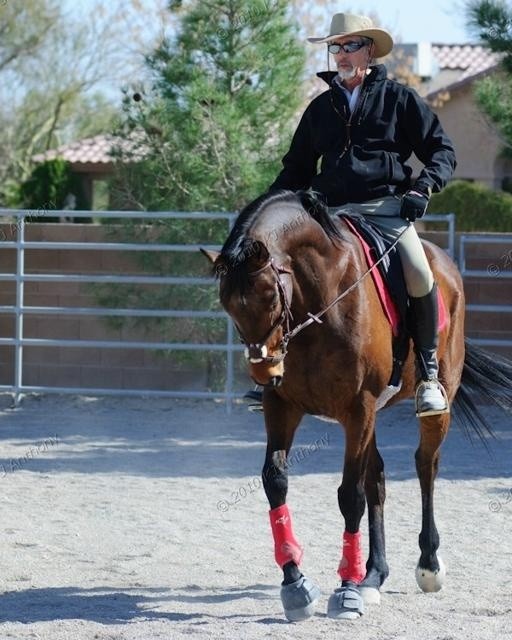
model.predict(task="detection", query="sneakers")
[242,382,262,406]
[411,376,451,419]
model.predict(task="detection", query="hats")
[306,12,394,58]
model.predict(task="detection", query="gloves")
[398,188,428,222]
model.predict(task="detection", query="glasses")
[327,40,369,53]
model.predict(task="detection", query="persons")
[245,12,458,418]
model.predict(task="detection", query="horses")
[199,188,512,621]
